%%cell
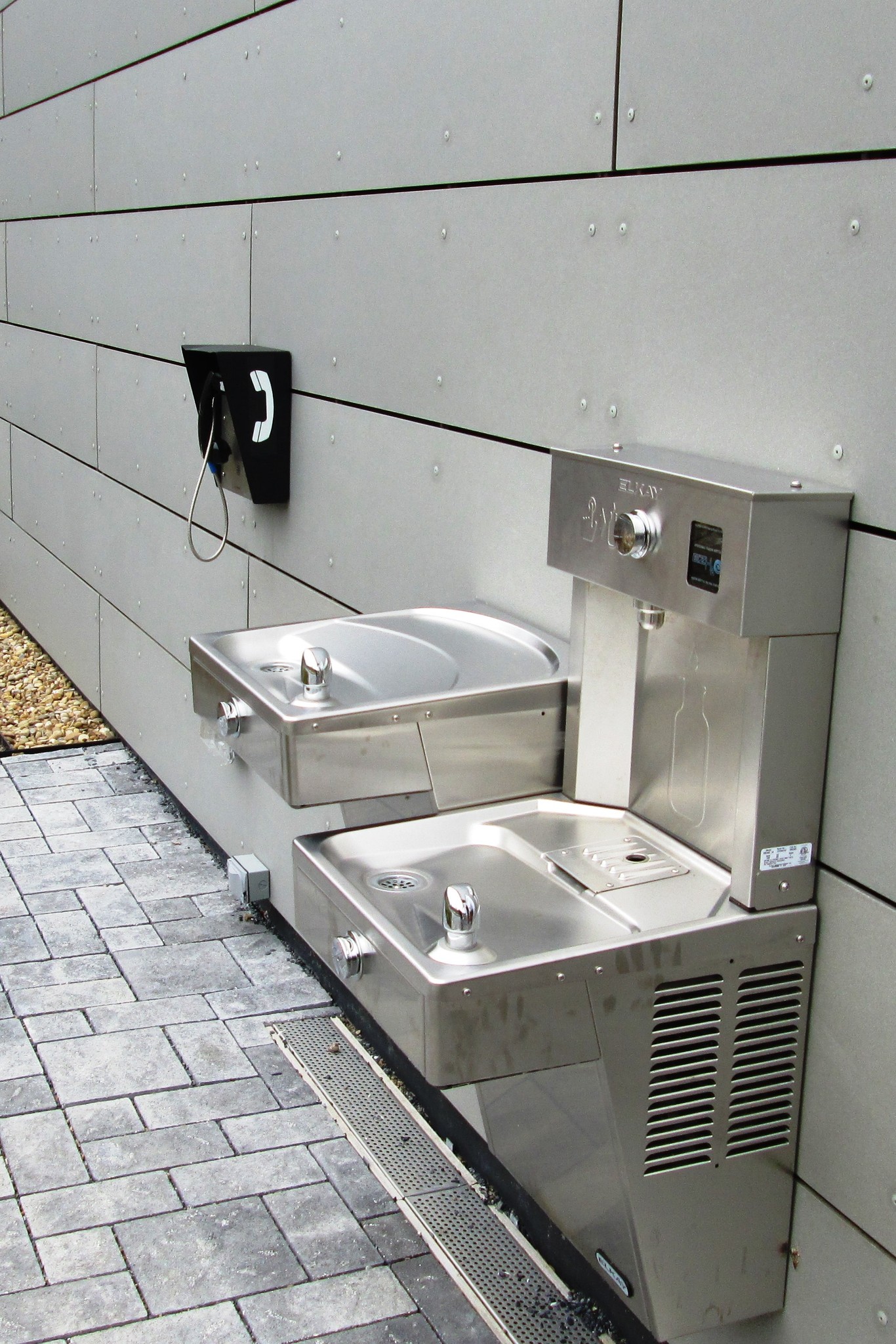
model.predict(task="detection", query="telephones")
[181,345,290,504]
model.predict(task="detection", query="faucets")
[631,599,665,630]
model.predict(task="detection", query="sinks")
[291,815,814,1089]
[188,600,569,809]
